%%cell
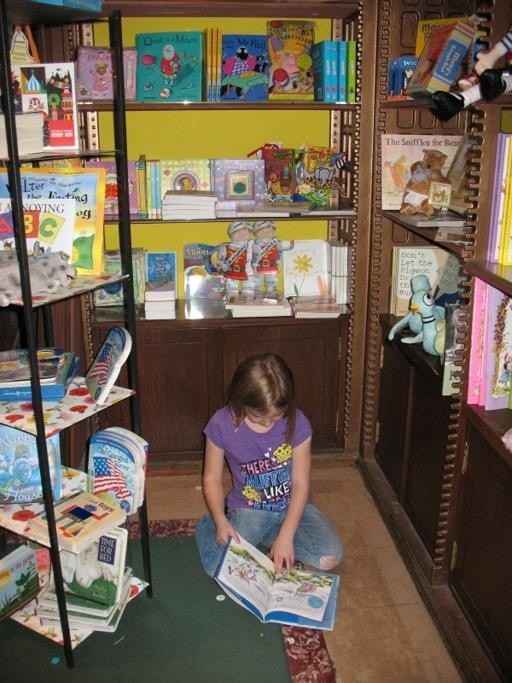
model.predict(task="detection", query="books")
[0,29,357,318]
[380,14,512,412]
[0,327,149,633]
[213,532,339,631]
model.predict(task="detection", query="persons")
[218,222,260,304]
[194,352,344,579]
[253,221,294,305]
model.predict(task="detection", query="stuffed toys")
[389,275,445,356]
[475,33,512,103]
[1,240,77,287]
[0,255,61,307]
[400,148,447,217]
[430,76,482,122]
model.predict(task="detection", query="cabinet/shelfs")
[364,0,507,683]
[41,0,359,471]
[0,1,150,667]
